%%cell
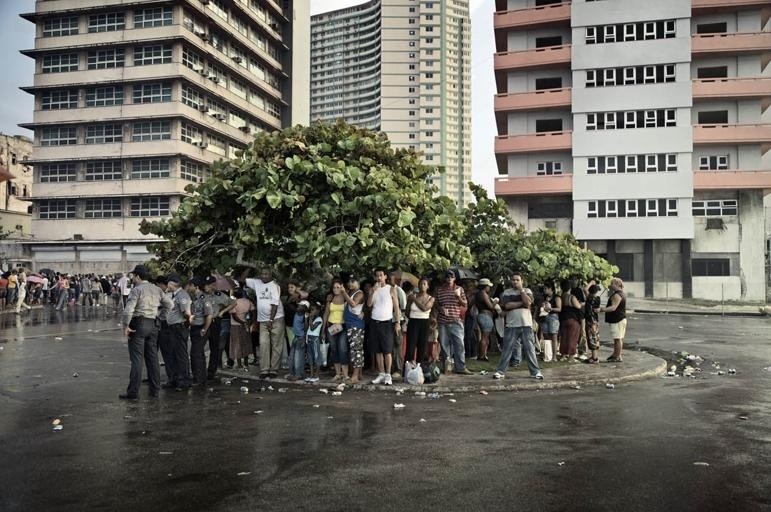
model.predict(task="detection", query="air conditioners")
[200,33,242,148]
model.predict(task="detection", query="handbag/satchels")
[246,310,257,320]
[320,339,330,366]
[328,323,343,336]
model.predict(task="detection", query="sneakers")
[216,359,258,372]
[493,372,506,378]
[535,346,622,363]
[439,357,488,375]
[530,372,543,379]
[260,365,392,384]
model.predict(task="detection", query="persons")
[118,264,626,397]
[1,267,136,314]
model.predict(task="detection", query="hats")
[479,278,493,287]
[446,270,454,275]
[134,265,216,285]
[298,300,310,309]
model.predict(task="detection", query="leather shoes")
[119,376,214,399]
